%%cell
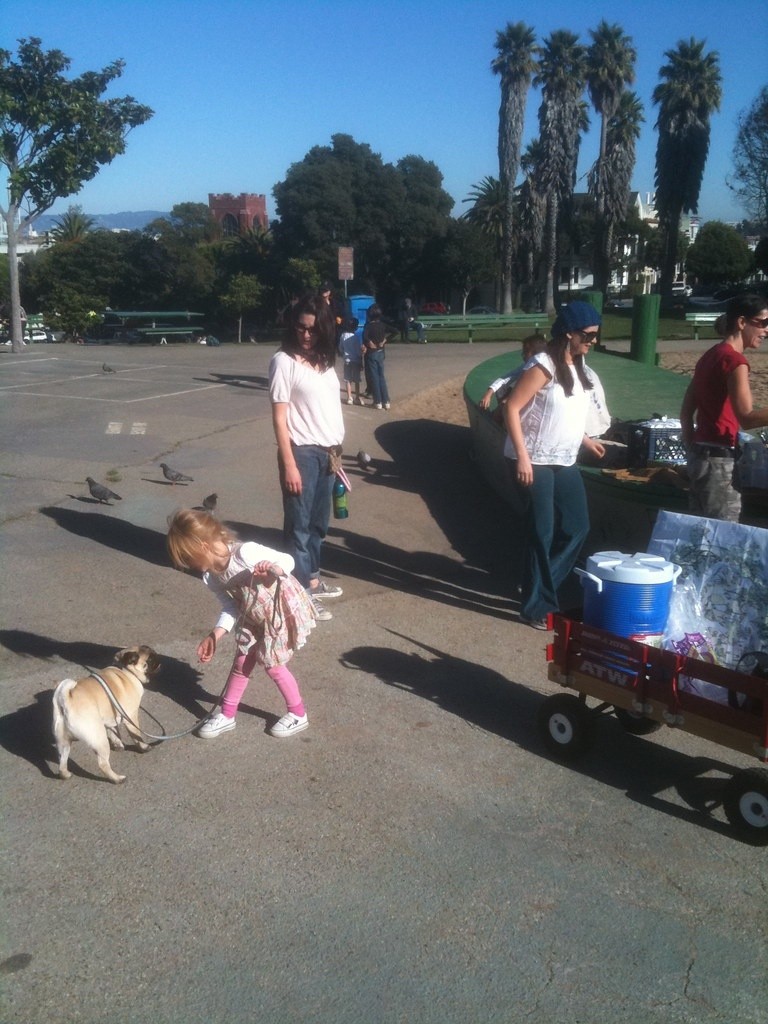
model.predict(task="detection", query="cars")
[672,281,693,297]
[24,332,57,343]
[418,300,450,316]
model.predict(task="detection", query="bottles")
[332,476,348,519]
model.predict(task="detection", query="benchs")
[399,312,552,344]
[23,314,66,342]
[685,312,726,340]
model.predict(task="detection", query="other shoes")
[355,398,365,406]
[418,338,427,343]
[346,396,353,405]
[374,401,383,410]
[382,401,391,410]
[518,612,548,629]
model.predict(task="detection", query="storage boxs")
[734,442,768,491]
[629,422,689,467]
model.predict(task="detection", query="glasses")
[745,315,768,328]
[578,328,601,342]
[295,322,319,337]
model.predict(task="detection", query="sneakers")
[304,588,333,620]
[198,711,236,738]
[269,711,309,737]
[310,581,343,598]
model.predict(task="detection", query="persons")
[479,334,547,409]
[679,296,768,523]
[362,304,390,410]
[339,317,364,405]
[168,509,313,740]
[319,286,342,324]
[402,298,427,343]
[502,300,605,630]
[269,295,345,620]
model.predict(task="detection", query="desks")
[104,323,204,345]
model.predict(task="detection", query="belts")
[689,441,738,459]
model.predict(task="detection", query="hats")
[551,301,602,336]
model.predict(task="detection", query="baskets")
[625,424,691,466]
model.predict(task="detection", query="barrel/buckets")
[574,550,683,648]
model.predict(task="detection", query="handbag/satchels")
[328,445,344,474]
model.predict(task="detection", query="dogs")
[53,645,164,784]
